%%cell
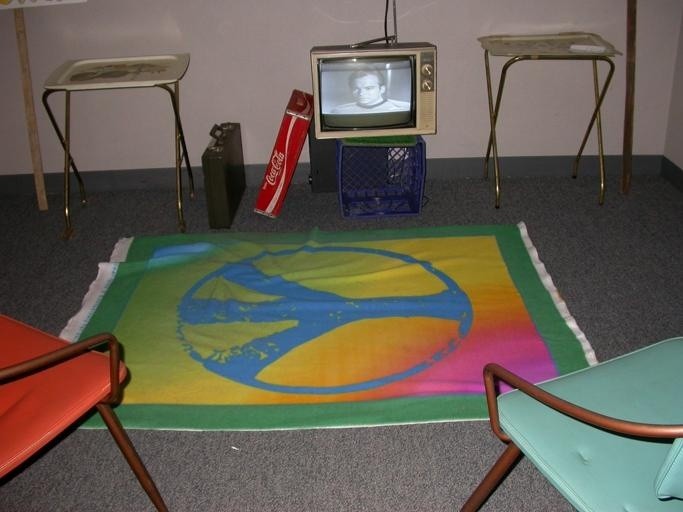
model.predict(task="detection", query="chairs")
[0,311,166,511]
[453,332,682,510]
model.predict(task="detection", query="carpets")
[57,217,600,436]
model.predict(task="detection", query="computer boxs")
[308,113,388,193]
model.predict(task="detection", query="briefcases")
[202,122,245,228]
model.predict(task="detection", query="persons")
[329,65,411,116]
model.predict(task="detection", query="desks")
[478,29,624,209]
[41,50,196,231]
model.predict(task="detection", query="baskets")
[336,135,428,219]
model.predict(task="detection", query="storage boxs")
[253,89,313,219]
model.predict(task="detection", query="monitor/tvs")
[309,41,436,139]
